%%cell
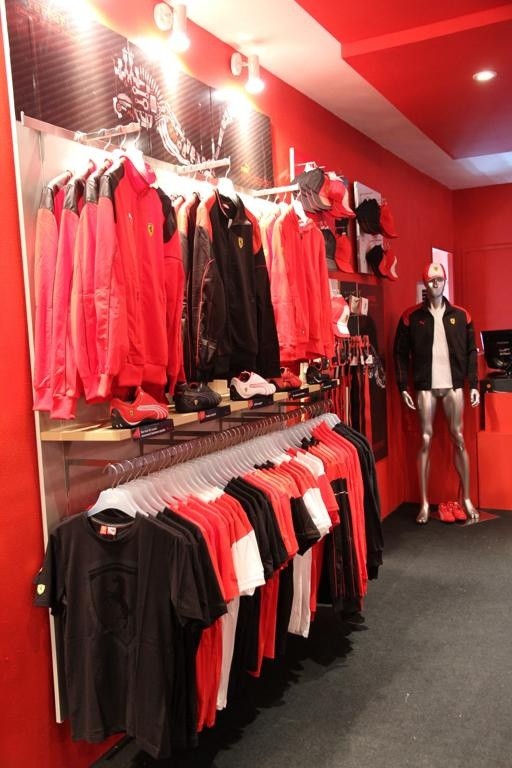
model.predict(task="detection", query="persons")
[393,261,481,524]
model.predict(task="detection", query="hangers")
[165,156,246,219]
[335,331,382,368]
[65,119,158,184]
[83,399,344,522]
[256,178,314,227]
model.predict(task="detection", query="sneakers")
[306,361,329,384]
[438,502,456,522]
[446,500,467,520]
[230,370,276,400]
[174,381,222,412]
[109,387,169,428]
[269,366,302,391]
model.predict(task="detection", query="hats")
[423,262,447,281]
[289,167,400,281]
[331,295,351,337]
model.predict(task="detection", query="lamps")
[152,1,194,58]
[229,49,268,97]
